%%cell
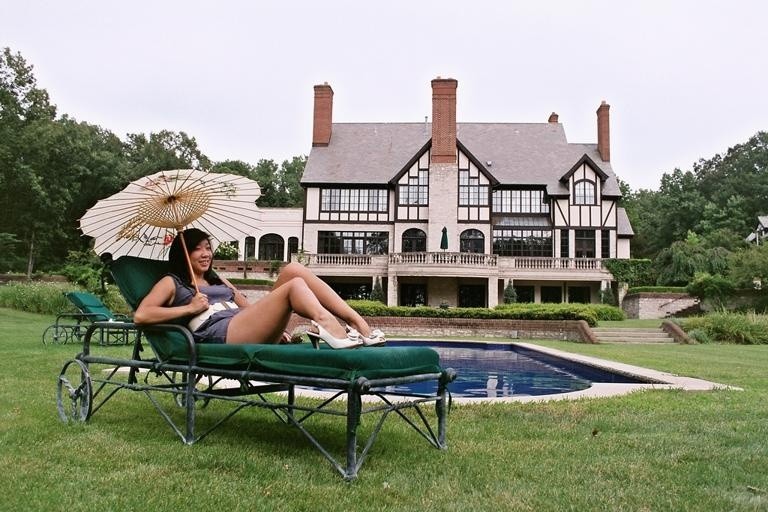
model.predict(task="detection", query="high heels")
[306,320,363,349]
[346,323,385,346]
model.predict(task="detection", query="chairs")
[56,256,456,482]
[42,292,147,346]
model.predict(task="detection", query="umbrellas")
[440,226,448,251]
[76,167,265,294]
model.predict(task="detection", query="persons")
[133,228,386,350]
